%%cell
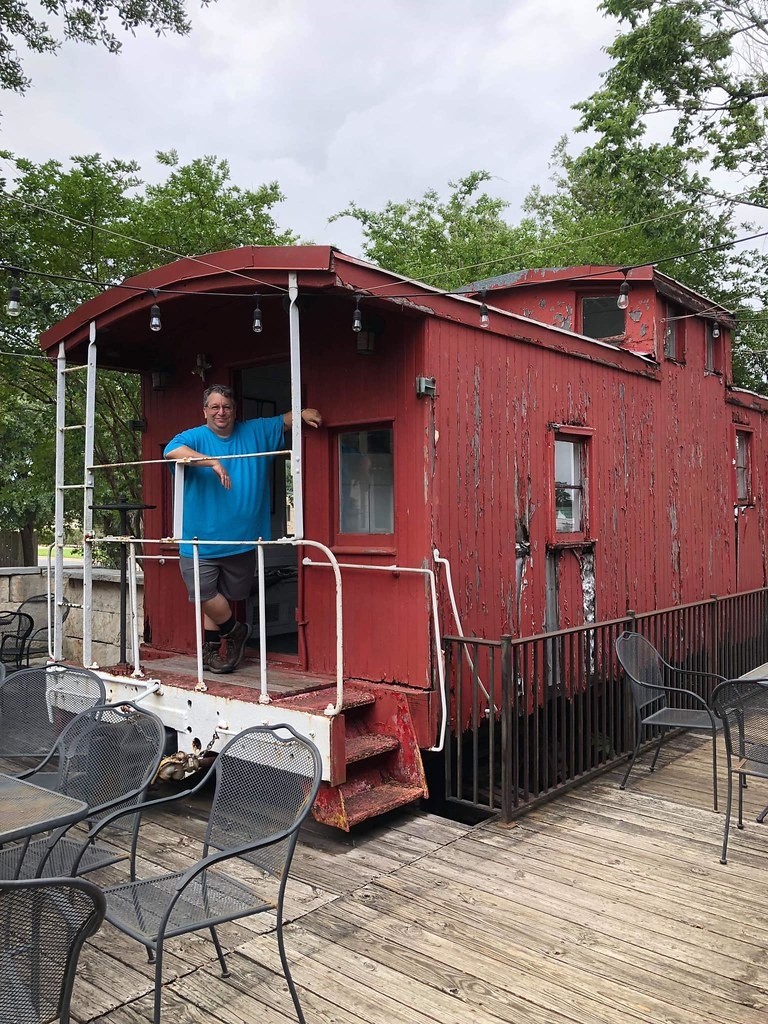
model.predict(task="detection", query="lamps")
[148,357,168,390]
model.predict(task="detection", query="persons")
[163,383,324,673]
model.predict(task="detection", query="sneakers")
[202,642,235,673]
[219,621,252,665]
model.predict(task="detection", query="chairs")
[0,591,323,1024]
[615,632,768,863]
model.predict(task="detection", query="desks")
[0,769,89,1010]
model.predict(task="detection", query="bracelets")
[301,407,307,412]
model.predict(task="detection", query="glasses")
[207,404,234,413]
